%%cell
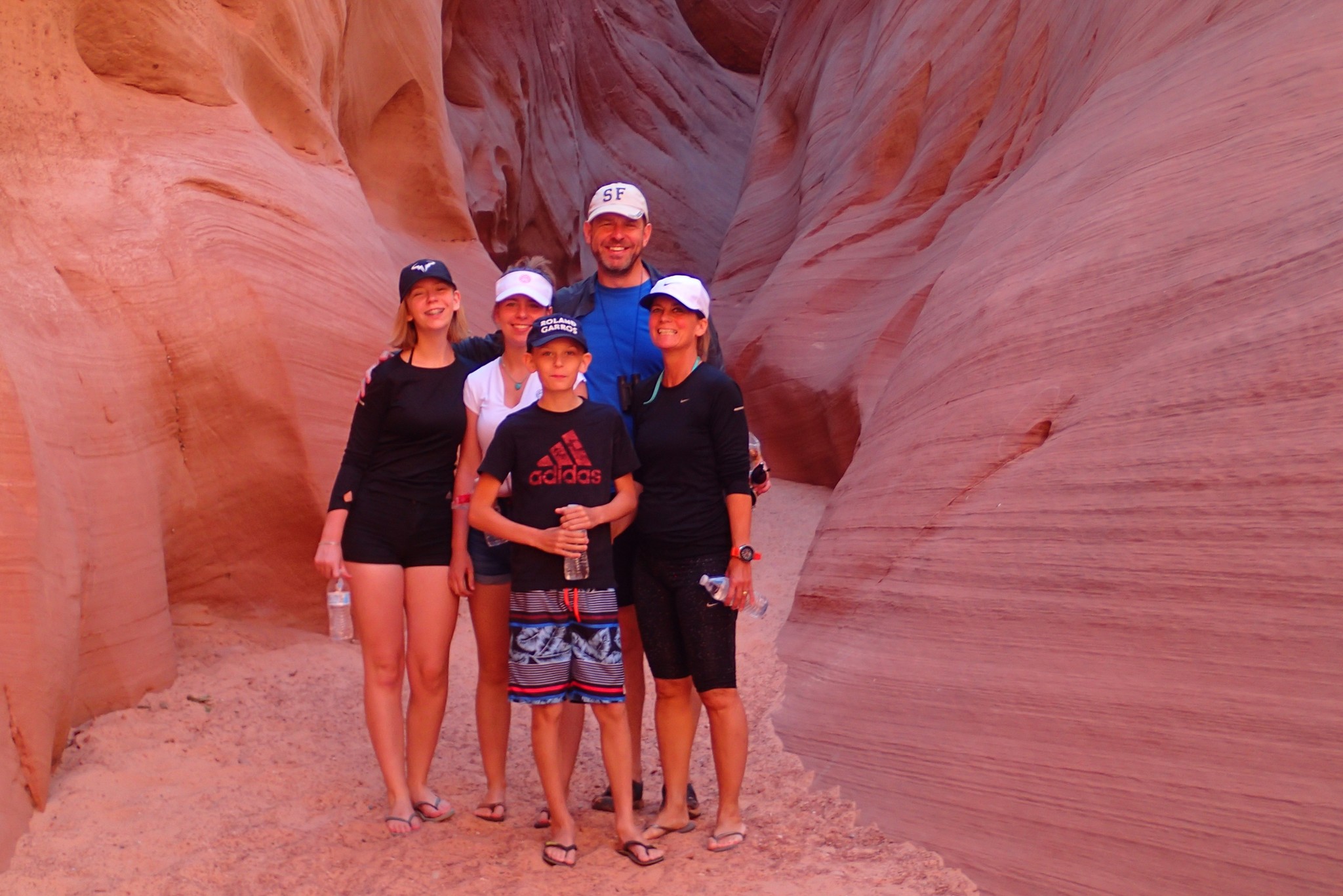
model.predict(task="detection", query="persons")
[619,273,753,851]
[448,254,588,827]
[313,258,509,837]
[473,310,667,867]
[358,182,770,854]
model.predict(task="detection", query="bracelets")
[321,541,340,546]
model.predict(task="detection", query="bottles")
[475,478,509,547]
[564,504,590,580]
[698,574,770,619]
[326,569,354,642]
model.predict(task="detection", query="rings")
[742,590,747,594]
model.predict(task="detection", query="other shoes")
[659,784,702,817]
[592,781,647,809]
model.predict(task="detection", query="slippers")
[616,840,663,866]
[385,811,422,836]
[639,823,696,840]
[542,841,578,867]
[707,830,747,852]
[412,795,454,821]
[535,806,553,827]
[476,801,507,820]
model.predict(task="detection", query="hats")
[398,259,457,304]
[587,182,649,225]
[495,270,553,306]
[640,275,710,318]
[526,313,587,353]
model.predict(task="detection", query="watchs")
[731,544,760,562]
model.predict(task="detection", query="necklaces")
[502,353,529,390]
